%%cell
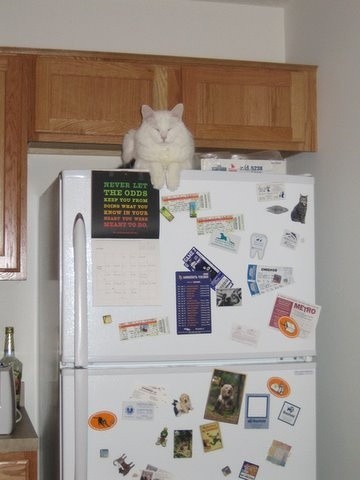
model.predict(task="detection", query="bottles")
[0,326,22,408]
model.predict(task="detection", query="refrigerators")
[58,170,317,480]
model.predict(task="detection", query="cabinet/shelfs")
[31,49,317,151]
[0,47,30,281]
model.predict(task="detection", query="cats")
[118,103,195,191]
